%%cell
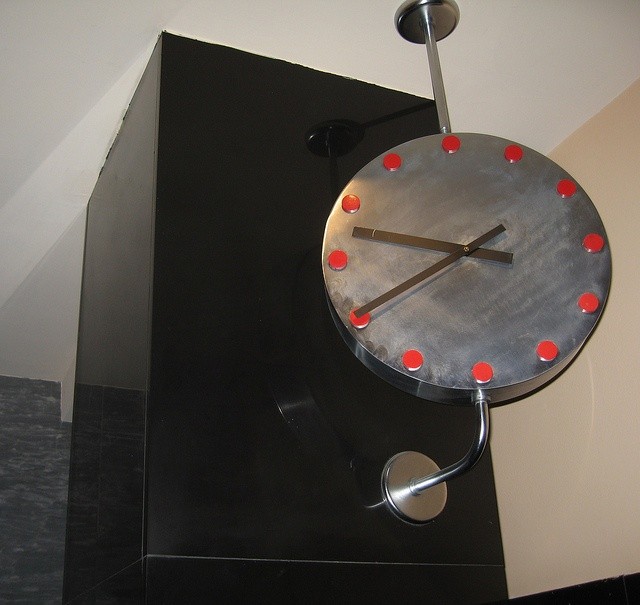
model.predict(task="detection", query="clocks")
[321,2,613,529]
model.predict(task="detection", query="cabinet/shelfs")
[65,29,515,604]
